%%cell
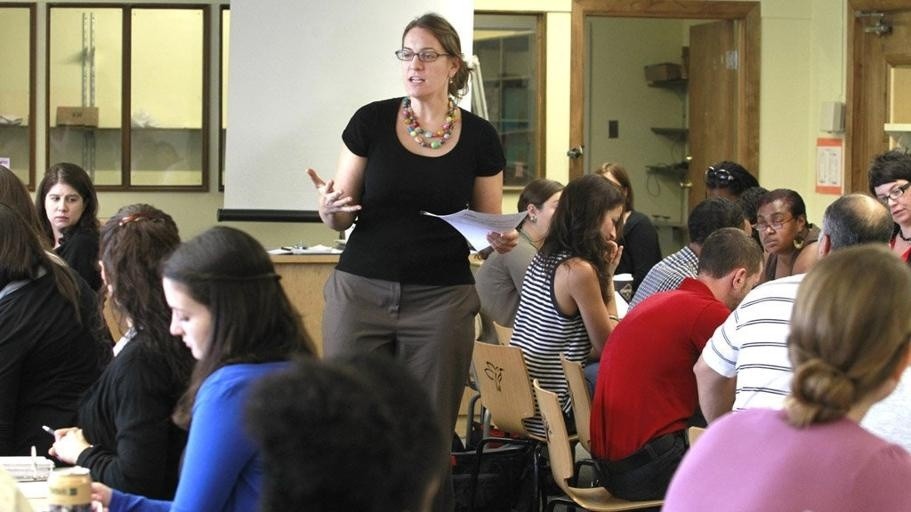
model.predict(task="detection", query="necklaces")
[402,96,458,150]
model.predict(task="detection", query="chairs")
[552,355,596,458]
[460,340,575,512]
[526,375,666,511]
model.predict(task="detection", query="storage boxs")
[644,61,682,81]
[56,104,98,128]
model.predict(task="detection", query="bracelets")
[608,312,619,322]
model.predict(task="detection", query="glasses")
[704,166,733,181]
[752,217,793,231]
[878,183,910,204]
[395,49,449,62]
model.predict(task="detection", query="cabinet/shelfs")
[473,29,545,190]
[642,74,691,177]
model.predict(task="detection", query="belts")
[590,434,675,475]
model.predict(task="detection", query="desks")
[267,248,353,364]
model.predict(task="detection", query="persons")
[48,203,199,502]
[91,225,318,511]
[243,350,450,511]
[692,192,895,438]
[594,162,663,303]
[507,174,624,439]
[627,198,753,313]
[736,187,770,222]
[705,161,760,202]
[660,246,911,512]
[867,150,911,266]
[34,162,102,292]
[758,188,824,287]
[1,164,115,456]
[475,178,565,345]
[589,226,764,499]
[306,14,504,433]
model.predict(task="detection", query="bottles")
[47,465,94,512]
